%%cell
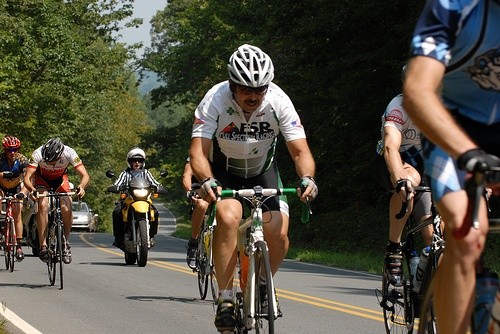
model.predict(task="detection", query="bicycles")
[374,180,447,334]
[185,182,220,304]
[417,154,500,334]
[33,185,84,290]
[204,182,313,334]
[0,192,29,273]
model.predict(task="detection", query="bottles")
[416,246,430,282]
[410,250,422,289]
[239,248,248,289]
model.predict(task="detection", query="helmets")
[227,44,274,87]
[1,136,20,148]
[126,147,145,169]
[41,137,64,162]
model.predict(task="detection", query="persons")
[188,44,319,334]
[374,0,500,334]
[0,136,209,268]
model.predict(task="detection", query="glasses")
[240,84,267,95]
[6,148,18,152]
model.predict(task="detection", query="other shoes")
[0,242,6,251]
[150,237,155,247]
[39,245,49,261]
[16,246,24,260]
[63,243,71,264]
[186,243,198,269]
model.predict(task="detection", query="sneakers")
[384,250,404,287]
[214,297,236,331]
[259,285,279,320]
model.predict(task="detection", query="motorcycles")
[105,169,169,267]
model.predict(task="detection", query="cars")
[71,202,100,233]
